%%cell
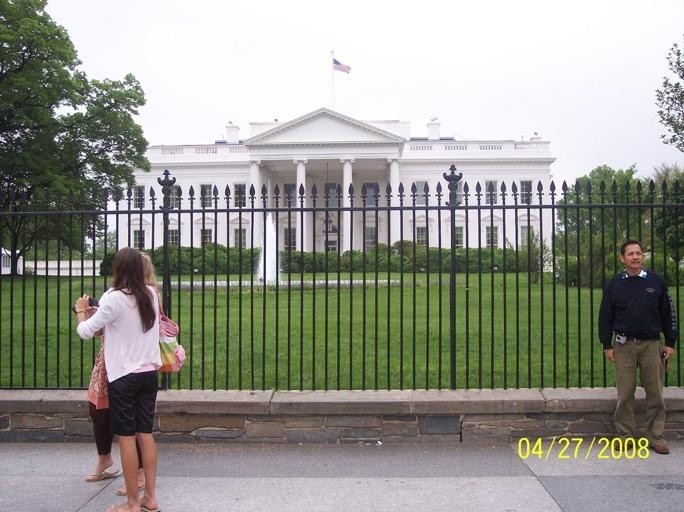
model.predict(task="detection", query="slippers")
[116,477,147,497]
[106,498,161,512]
[85,464,122,482]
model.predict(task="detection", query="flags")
[333,58,352,74]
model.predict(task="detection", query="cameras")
[72,297,98,315]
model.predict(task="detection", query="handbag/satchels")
[155,291,186,373]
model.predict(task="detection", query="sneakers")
[615,442,633,451]
[651,438,670,454]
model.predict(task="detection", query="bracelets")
[75,310,86,315]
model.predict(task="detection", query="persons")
[596,239,680,457]
[73,247,166,512]
[83,251,161,498]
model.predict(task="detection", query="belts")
[615,331,661,343]
